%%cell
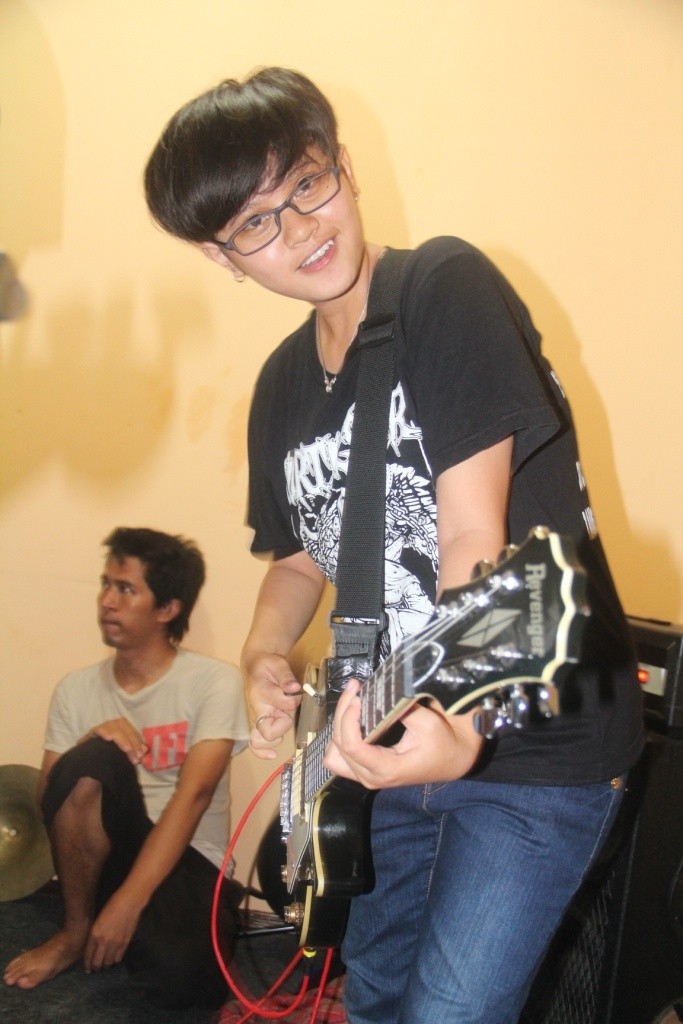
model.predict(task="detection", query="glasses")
[211,155,341,256]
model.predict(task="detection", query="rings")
[255,714,276,730]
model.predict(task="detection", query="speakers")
[519,612,683,1023]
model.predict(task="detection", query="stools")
[0,882,138,1024]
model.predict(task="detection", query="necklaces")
[314,239,372,394]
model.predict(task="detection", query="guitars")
[279,526,591,948]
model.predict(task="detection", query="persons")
[144,65,647,1024]
[3,528,256,1023]
